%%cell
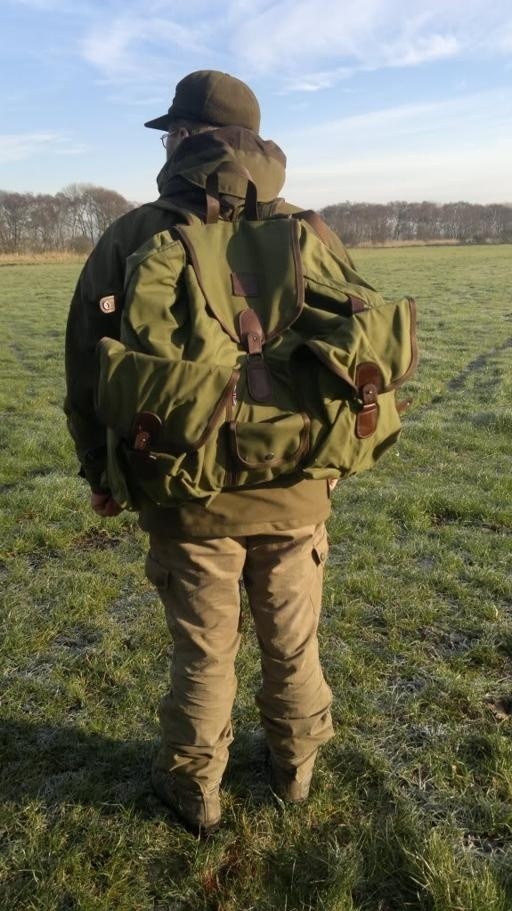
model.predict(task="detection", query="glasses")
[160,127,181,147]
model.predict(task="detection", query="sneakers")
[270,756,310,800]
[151,748,221,836]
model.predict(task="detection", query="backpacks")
[92,160,415,510]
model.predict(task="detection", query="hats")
[145,71,260,132]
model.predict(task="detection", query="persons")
[64,68,418,833]
